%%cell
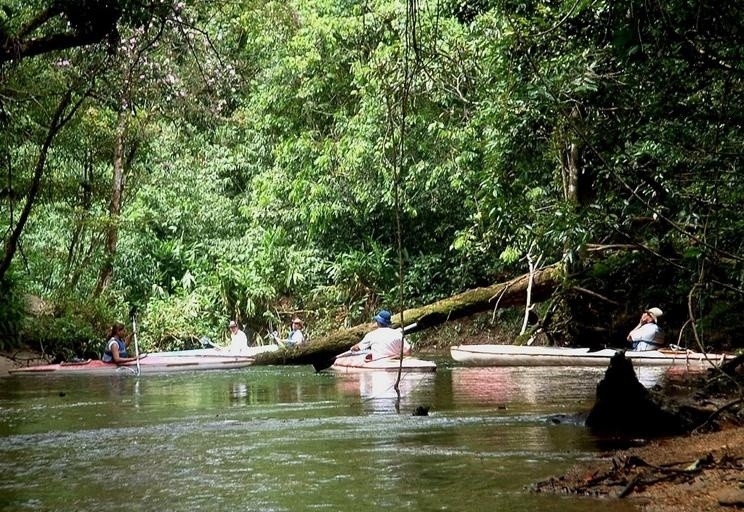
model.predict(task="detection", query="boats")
[450,344,737,366]
[8,344,278,377]
[334,354,437,372]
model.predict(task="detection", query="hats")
[291,317,304,328]
[370,310,393,327]
[643,306,664,324]
[227,320,237,327]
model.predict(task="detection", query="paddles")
[133,314,143,378]
[311,312,446,373]
[200,335,229,352]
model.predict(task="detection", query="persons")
[100,322,137,365]
[352,369,412,414]
[214,319,247,352]
[275,318,304,348]
[626,305,666,351]
[348,310,410,362]
[228,369,251,410]
